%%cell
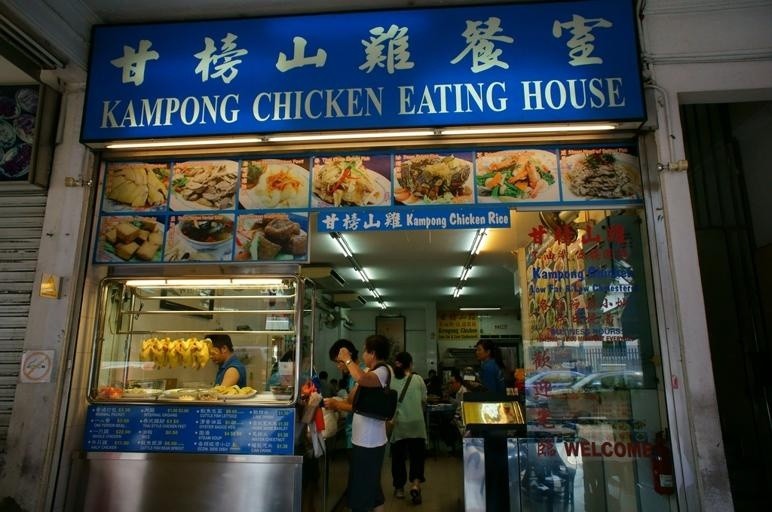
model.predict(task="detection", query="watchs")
[344,358,353,367]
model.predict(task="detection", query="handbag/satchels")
[385,373,415,439]
[350,363,398,422]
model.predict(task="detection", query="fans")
[319,310,338,330]
[537,212,596,246]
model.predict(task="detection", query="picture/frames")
[375,316,406,362]
[160,289,216,319]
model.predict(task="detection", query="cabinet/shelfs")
[583,389,672,512]
[86,276,316,409]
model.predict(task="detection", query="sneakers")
[393,488,406,499]
[410,484,423,505]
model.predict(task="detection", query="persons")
[330,379,338,389]
[337,334,394,512]
[425,369,437,386]
[474,337,507,405]
[203,329,247,388]
[317,369,338,398]
[441,374,467,452]
[321,339,368,469]
[392,351,427,504]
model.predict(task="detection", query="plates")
[209,389,257,399]
[122,389,163,398]
[164,389,208,399]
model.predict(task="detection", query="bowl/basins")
[177,390,199,401]
[198,390,218,401]
[271,386,294,401]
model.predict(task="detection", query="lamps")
[460,267,467,281]
[124,280,166,284]
[380,303,384,309]
[167,279,231,283]
[441,125,617,138]
[464,268,471,280]
[370,290,376,299]
[268,131,439,144]
[454,288,458,298]
[382,303,386,309]
[457,288,461,298]
[476,228,490,255]
[459,307,500,311]
[356,270,365,283]
[361,269,370,283]
[471,228,483,255]
[374,289,379,298]
[230,278,282,284]
[332,238,347,257]
[338,237,353,258]
[106,137,264,151]
